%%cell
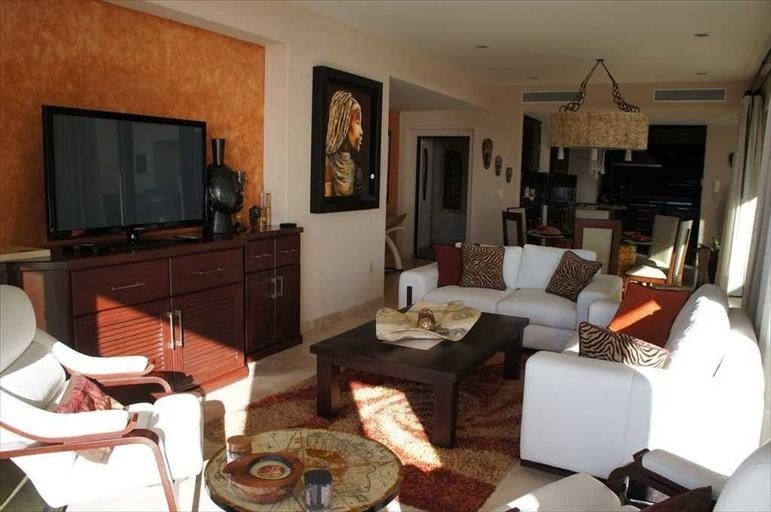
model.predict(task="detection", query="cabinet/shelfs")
[70,246,246,394]
[246,232,302,362]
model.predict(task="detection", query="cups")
[259,192,272,227]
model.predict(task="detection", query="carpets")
[201,346,543,512]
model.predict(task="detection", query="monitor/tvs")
[43,103,207,252]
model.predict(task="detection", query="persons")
[324,90,364,196]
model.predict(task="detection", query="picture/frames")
[310,65,384,213]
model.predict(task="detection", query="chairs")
[504,201,694,291]
[0,283,208,512]
[519,281,766,488]
[487,440,771,512]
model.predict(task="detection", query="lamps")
[545,60,650,180]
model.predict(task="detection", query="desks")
[205,425,405,512]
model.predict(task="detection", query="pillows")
[606,282,693,348]
[42,369,127,464]
[432,242,482,288]
[577,321,669,368]
[546,250,603,303]
[638,484,713,511]
[458,241,507,290]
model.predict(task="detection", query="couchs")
[398,240,624,356]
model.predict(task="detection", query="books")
[0,244,51,263]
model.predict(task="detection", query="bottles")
[227,435,252,462]
[304,469,332,511]
